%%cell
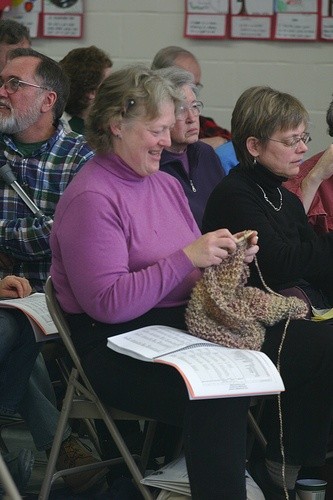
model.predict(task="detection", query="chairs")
[39,275,269,500]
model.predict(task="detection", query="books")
[1,292,61,342]
[102,323,285,404]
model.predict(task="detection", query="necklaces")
[256,183,283,211]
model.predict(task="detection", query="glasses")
[0,77,50,94]
[261,131,312,148]
[195,83,203,97]
[174,100,204,121]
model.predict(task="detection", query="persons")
[47,65,333,500]
[57,46,115,137]
[285,98,333,236]
[0,20,32,75]
[0,50,99,292]
[149,47,202,90]
[0,272,107,495]
[151,70,228,234]
[202,83,333,330]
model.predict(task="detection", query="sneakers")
[47,434,112,496]
